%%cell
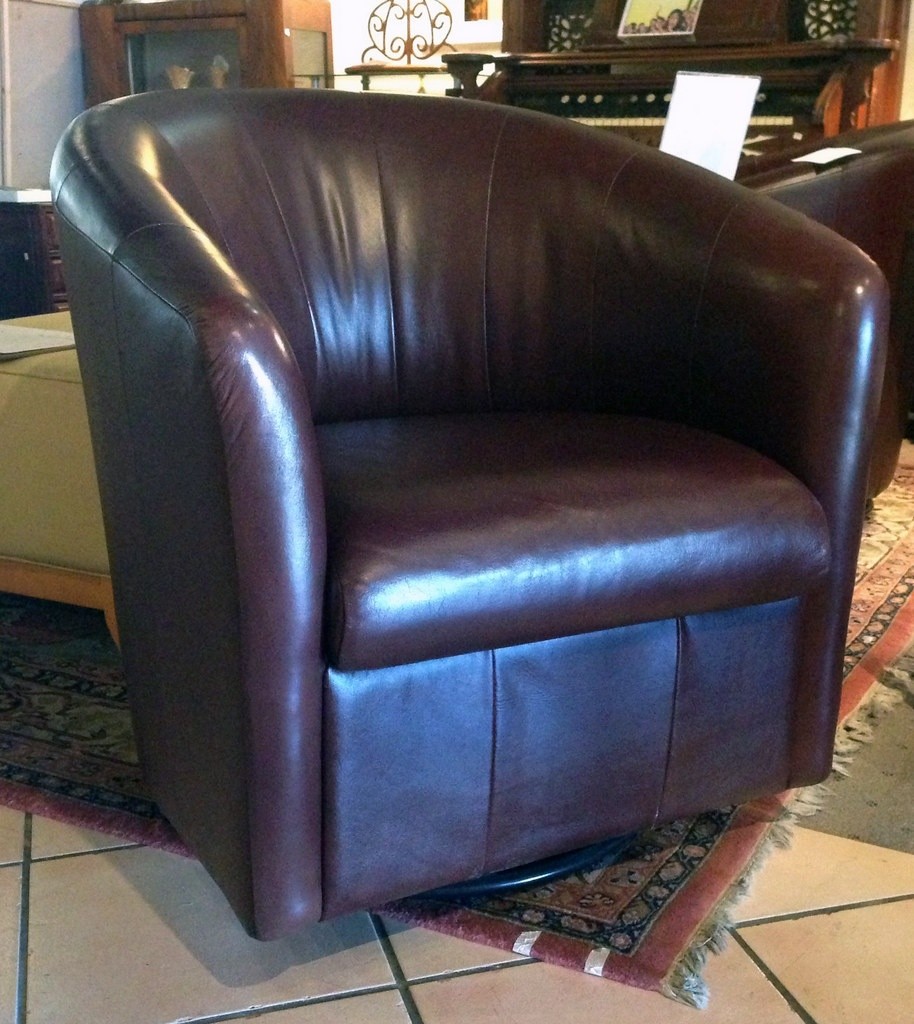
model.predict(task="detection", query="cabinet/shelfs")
[0,201,71,319]
[80,0,334,108]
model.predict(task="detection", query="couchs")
[0,89,914,941]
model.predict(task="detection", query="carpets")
[0,457,914,1012]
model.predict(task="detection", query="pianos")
[441,0,903,156]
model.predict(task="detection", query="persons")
[622,8,698,34]
